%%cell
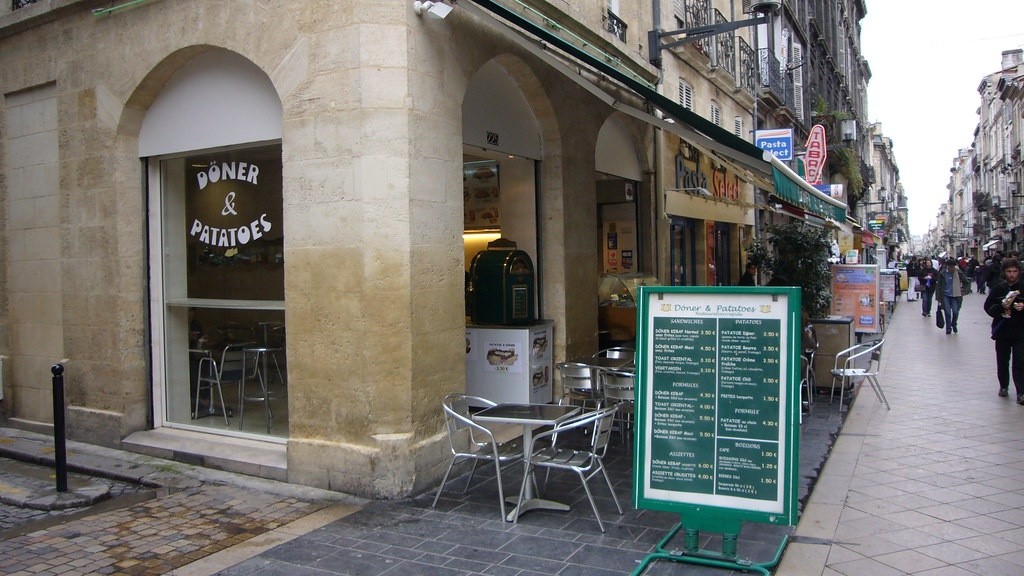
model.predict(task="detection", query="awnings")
[461,0,883,247]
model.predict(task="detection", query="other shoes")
[922,311,932,317]
[998,384,1008,397]
[1017,393,1024,404]
[952,324,957,333]
[947,328,951,334]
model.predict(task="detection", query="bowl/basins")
[610,295,619,300]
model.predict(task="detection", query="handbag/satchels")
[936,304,944,328]
[915,284,926,293]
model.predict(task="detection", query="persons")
[983,258,1024,403]
[739,262,756,286]
[905,251,1024,334]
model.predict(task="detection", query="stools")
[239,343,286,434]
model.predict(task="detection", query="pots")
[216,327,254,341]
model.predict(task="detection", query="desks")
[566,357,633,434]
[189,344,243,419]
[471,402,580,522]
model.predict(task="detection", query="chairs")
[551,344,638,443]
[799,342,820,425]
[195,320,287,426]
[512,407,624,534]
[830,339,890,412]
[430,394,540,523]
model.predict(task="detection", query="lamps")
[982,211,996,221]
[946,231,958,238]
[953,227,964,234]
[1009,182,1024,198]
[648,0,782,69]
[990,194,1018,211]
[794,119,857,157]
[413,1,453,20]
[962,219,973,228]
[680,143,689,158]
[665,187,713,198]
[857,187,887,207]
